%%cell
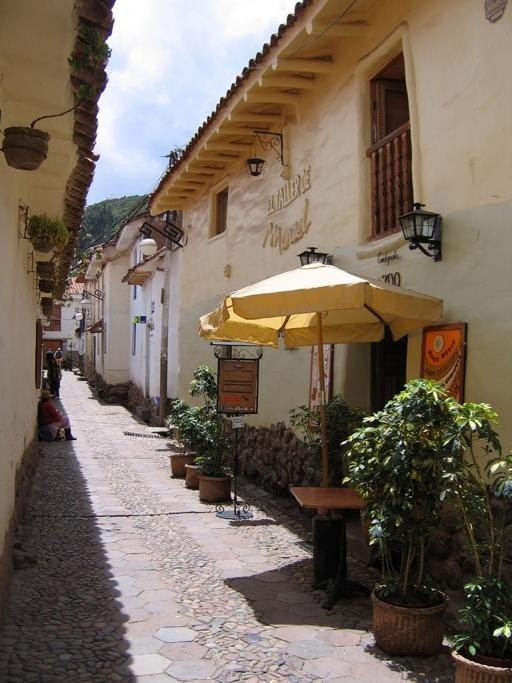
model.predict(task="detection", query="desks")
[289,485,369,608]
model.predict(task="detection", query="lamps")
[79,289,103,309]
[295,245,331,266]
[245,128,284,176]
[393,199,444,264]
[137,220,184,255]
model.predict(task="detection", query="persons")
[56,371,63,398]
[43,358,60,399]
[54,347,63,369]
[38,390,77,441]
[46,348,54,376]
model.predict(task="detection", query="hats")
[39,391,54,399]
[58,348,61,350]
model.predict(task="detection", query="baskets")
[371,583,450,656]
[451,642,512,682]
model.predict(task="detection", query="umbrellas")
[197,259,445,487]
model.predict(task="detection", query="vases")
[35,259,56,316]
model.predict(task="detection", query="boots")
[65,428,76,440]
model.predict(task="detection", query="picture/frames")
[418,322,467,404]
[306,343,335,434]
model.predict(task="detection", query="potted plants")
[164,362,234,505]
[1,25,111,170]
[26,213,69,252]
[442,461,511,681]
[344,376,500,662]
